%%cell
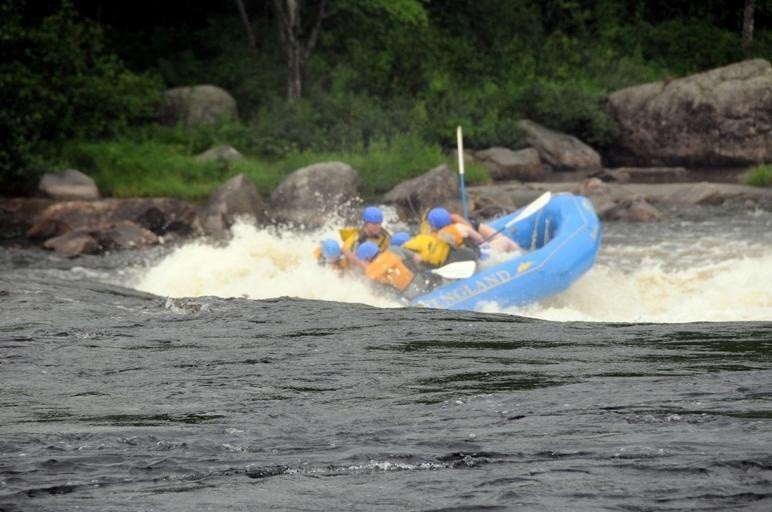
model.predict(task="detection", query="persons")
[320,205,539,298]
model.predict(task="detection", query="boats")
[403,192,600,313]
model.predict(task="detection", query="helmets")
[391,232,409,246]
[428,208,451,229]
[322,240,340,258]
[363,207,382,222]
[357,242,379,260]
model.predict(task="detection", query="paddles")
[457,125,467,221]
[423,261,475,278]
[475,191,551,247]
